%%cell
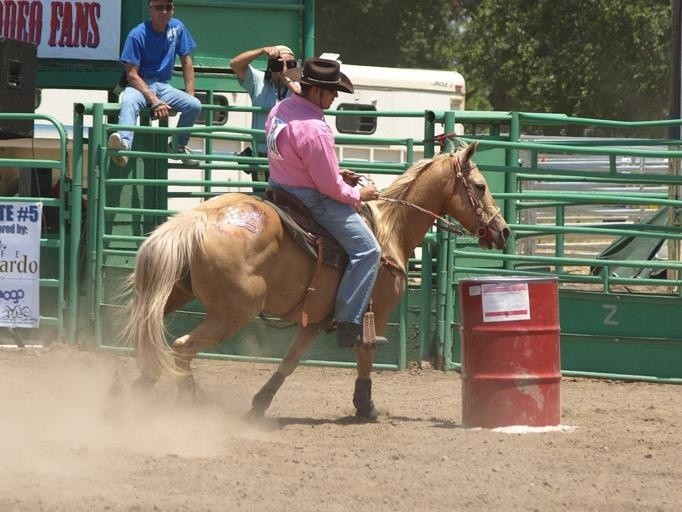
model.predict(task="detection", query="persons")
[107,1,204,169]
[264,56,389,349]
[228,40,301,197]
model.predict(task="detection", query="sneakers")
[107,131,130,168]
[336,321,390,348]
[165,139,201,167]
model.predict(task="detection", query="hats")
[266,44,295,72]
[281,57,355,95]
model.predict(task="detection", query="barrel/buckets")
[458,275,563,430]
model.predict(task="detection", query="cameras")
[268,55,297,72]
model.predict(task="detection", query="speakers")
[1,38,37,137]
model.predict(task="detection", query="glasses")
[153,4,172,11]
